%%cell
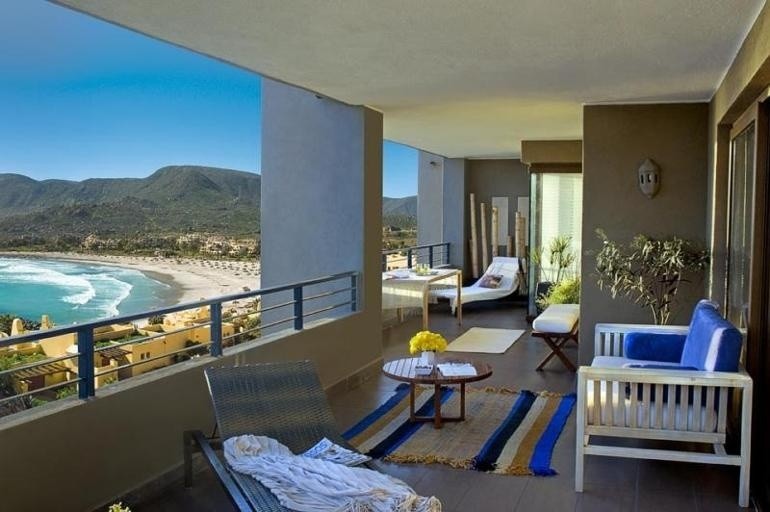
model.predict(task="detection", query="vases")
[422,351,434,365]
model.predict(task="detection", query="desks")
[382,357,493,429]
[382,268,462,331]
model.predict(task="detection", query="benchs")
[531,304,579,373]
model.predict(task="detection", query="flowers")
[409,331,447,353]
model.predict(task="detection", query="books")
[436,362,478,377]
[300,436,373,467]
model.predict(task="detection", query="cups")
[416,263,430,275]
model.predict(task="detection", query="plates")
[416,364,433,375]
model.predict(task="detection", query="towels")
[436,363,476,376]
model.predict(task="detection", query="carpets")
[444,325,526,353]
[340,383,577,476]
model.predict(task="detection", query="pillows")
[479,274,503,289]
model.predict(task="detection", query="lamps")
[639,158,660,199]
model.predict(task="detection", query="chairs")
[428,257,526,317]
[192,361,382,512]
[574,299,754,507]
